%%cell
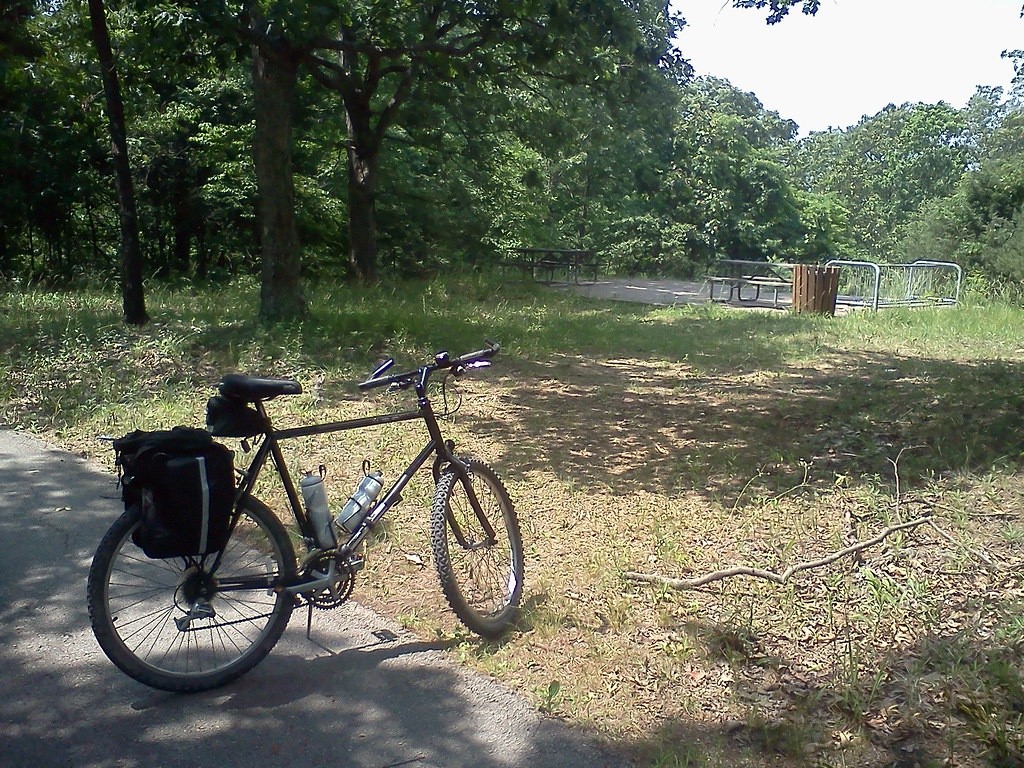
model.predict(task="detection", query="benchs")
[495,258,607,273]
[702,274,798,287]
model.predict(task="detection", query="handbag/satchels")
[134,444,236,558]
[113,426,213,546]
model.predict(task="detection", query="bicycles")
[85,338,526,693]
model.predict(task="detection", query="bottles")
[338,469,384,534]
[300,469,339,552]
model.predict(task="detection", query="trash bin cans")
[793,263,841,318]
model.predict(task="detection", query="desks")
[507,248,598,288]
[718,259,821,308]
